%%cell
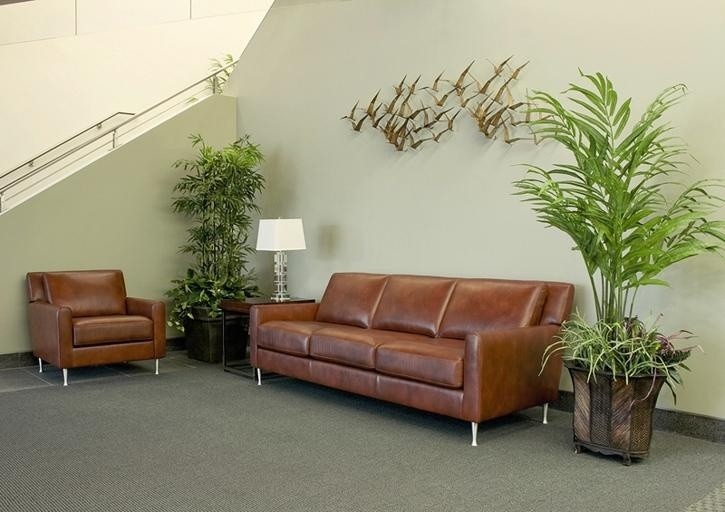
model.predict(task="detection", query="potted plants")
[164,133,270,363]
[510,68,724,466]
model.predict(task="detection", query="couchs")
[250,272,575,446]
[25,267,166,386]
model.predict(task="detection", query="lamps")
[256,215,307,301]
